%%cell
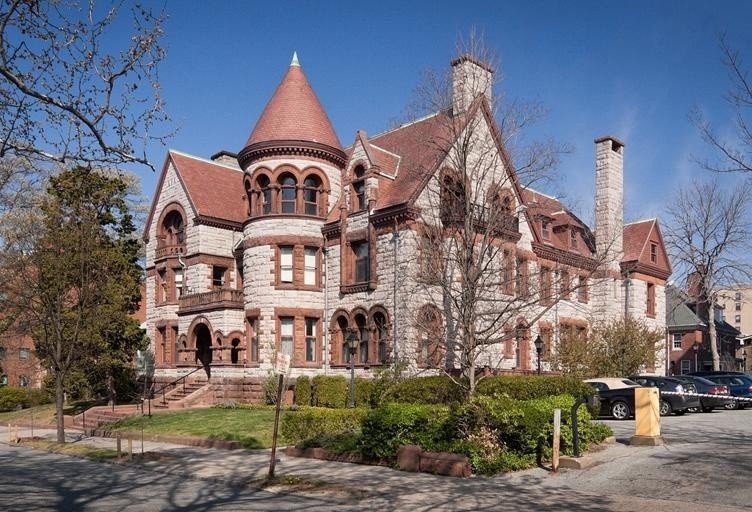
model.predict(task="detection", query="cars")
[701,375,751,410]
[582,377,662,419]
[671,376,733,413]
[626,375,700,416]
